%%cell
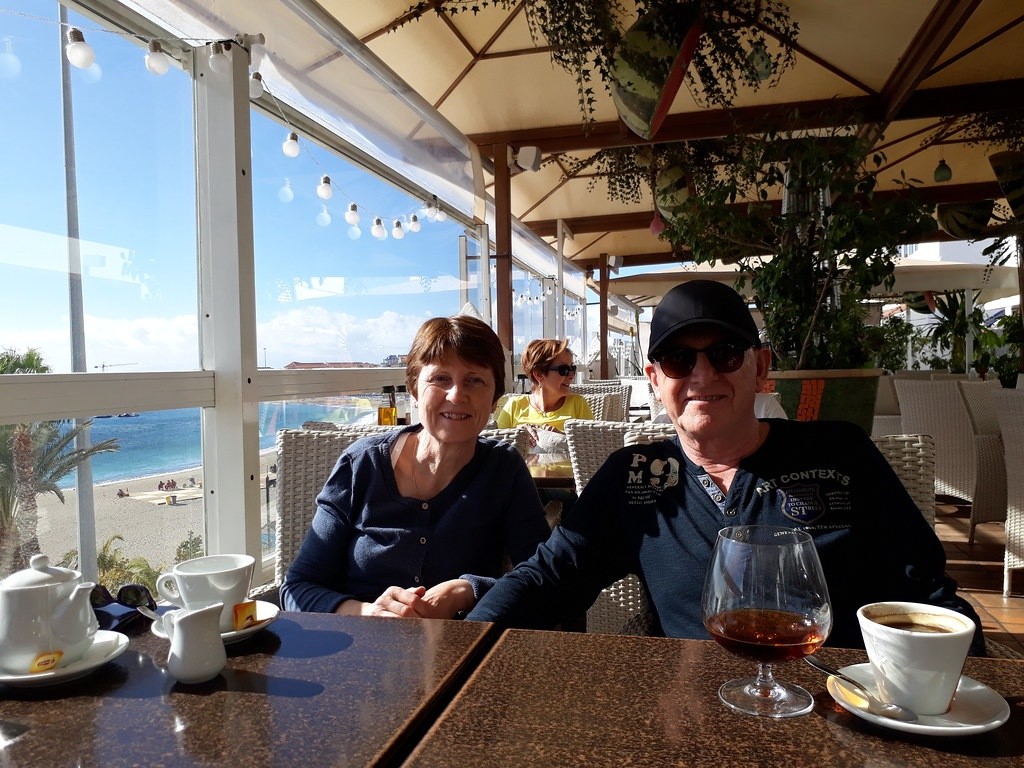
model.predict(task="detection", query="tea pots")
[0,553,99,676]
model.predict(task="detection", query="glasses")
[90,584,157,630]
[541,366,576,376]
[657,340,758,379]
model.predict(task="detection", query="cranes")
[93,363,138,372]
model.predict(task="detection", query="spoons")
[804,655,919,723]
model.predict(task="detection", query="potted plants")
[386,0,934,439]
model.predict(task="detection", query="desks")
[0,607,494,768]
[398,628,1024,768]
[522,451,578,490]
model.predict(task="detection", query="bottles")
[378,385,397,426]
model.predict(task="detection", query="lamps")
[507,145,542,173]
[608,256,622,276]
[748,41,772,81]
[935,143,953,183]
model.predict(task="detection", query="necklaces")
[410,434,422,501]
[547,397,563,411]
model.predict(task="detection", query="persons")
[461,279,988,658]
[278,316,552,621]
[170,479,176,488]
[485,338,593,436]
[165,480,170,489]
[117,489,129,498]
[158,481,165,490]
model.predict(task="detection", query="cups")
[155,553,256,633]
[856,601,976,716]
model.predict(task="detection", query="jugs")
[162,603,227,685]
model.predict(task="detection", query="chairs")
[875,371,1024,599]
[275,379,934,636]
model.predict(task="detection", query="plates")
[150,600,280,646]
[0,630,130,688]
[826,663,1011,736]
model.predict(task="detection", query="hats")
[648,280,759,363]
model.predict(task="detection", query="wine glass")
[700,524,833,718]
